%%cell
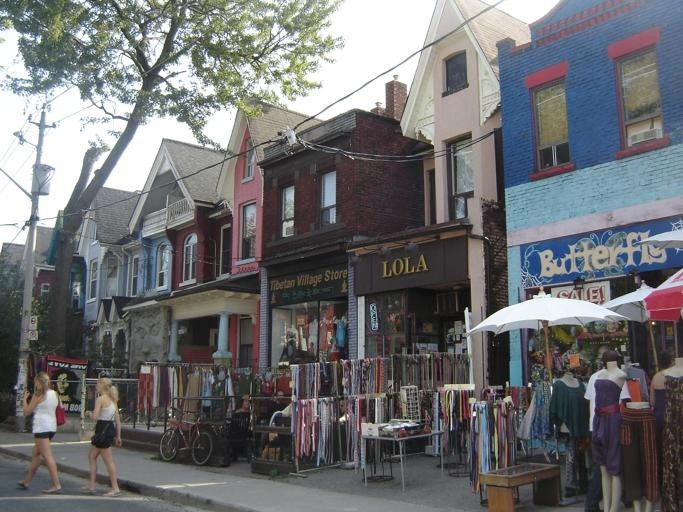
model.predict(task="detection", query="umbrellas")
[465,229,683,387]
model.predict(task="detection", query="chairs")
[223,411,253,464]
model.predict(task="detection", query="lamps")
[573,276,586,290]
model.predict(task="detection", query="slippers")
[40,489,61,494]
[80,487,97,494]
[103,491,121,496]
[18,480,29,488]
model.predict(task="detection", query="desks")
[360,429,445,492]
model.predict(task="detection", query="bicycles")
[160,406,214,465]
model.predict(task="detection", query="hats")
[241,395,251,400]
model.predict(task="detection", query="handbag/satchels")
[56,395,65,425]
[90,434,105,445]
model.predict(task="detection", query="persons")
[17,373,62,494]
[660,357,682,512]
[269,413,291,460]
[51,368,81,406]
[236,395,258,431]
[81,378,122,496]
[621,402,659,512]
[330,399,350,423]
[584,350,622,512]
[590,360,627,512]
[548,372,588,436]
[270,391,290,416]
